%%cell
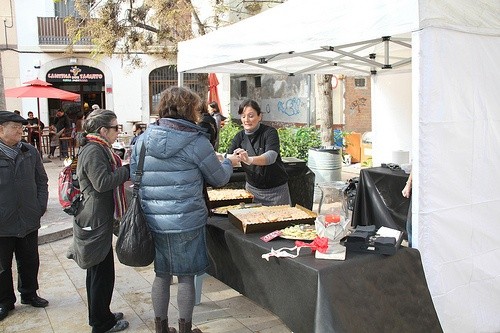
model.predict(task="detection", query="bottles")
[315,180,350,240]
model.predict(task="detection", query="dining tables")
[352,164,414,240]
[114,182,445,333]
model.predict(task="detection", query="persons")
[132,124,143,146]
[217,98,294,207]
[196,101,217,148]
[56,110,69,159]
[77,112,85,120]
[27,111,44,151]
[71,120,86,148]
[130,87,242,333]
[66,110,130,333]
[48,124,65,159]
[402,170,412,250]
[0,111,49,323]
[208,101,221,152]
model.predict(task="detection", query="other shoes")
[105,312,129,333]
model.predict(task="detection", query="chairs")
[22,125,74,160]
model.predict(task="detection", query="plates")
[204,187,254,209]
[210,203,260,215]
[227,204,319,235]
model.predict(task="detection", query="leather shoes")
[0,304,15,321]
[21,293,49,307]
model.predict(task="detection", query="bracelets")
[405,182,412,184]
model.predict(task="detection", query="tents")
[177,0,500,333]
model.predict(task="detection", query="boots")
[155,316,203,333]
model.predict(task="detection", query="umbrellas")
[3,77,82,154]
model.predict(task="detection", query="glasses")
[30,115,33,116]
[107,125,118,132]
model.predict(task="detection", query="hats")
[0,111,28,125]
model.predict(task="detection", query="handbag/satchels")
[115,193,155,267]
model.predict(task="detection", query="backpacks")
[59,143,92,216]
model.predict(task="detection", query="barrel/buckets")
[305,145,345,205]
